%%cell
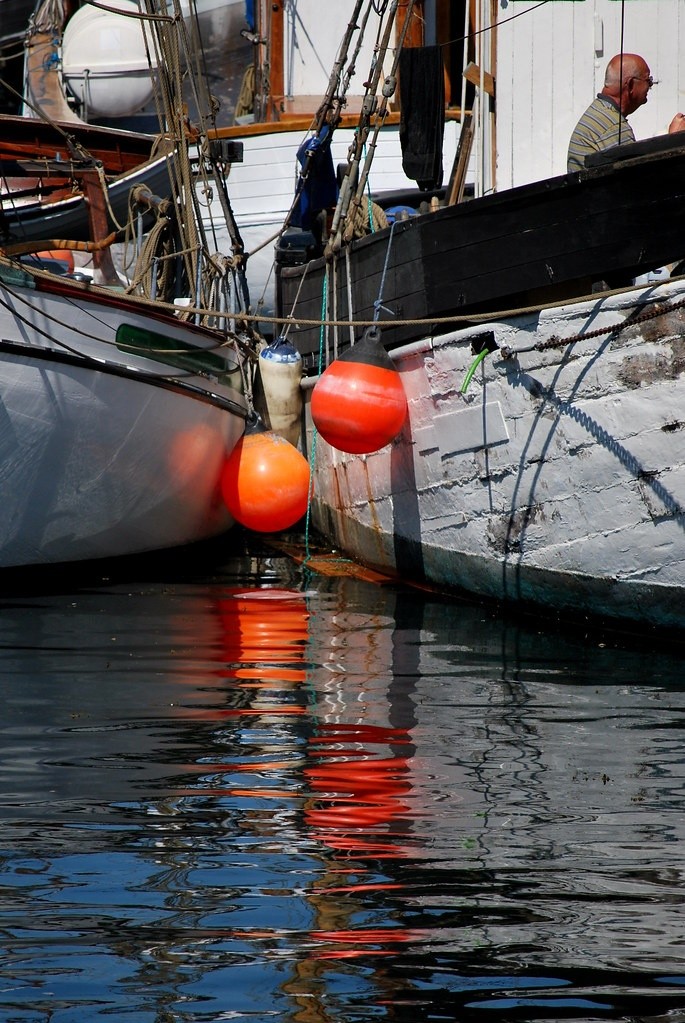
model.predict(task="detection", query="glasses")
[625,76,654,88]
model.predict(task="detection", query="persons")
[567,54,685,174]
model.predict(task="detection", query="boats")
[1,0,685,635]
